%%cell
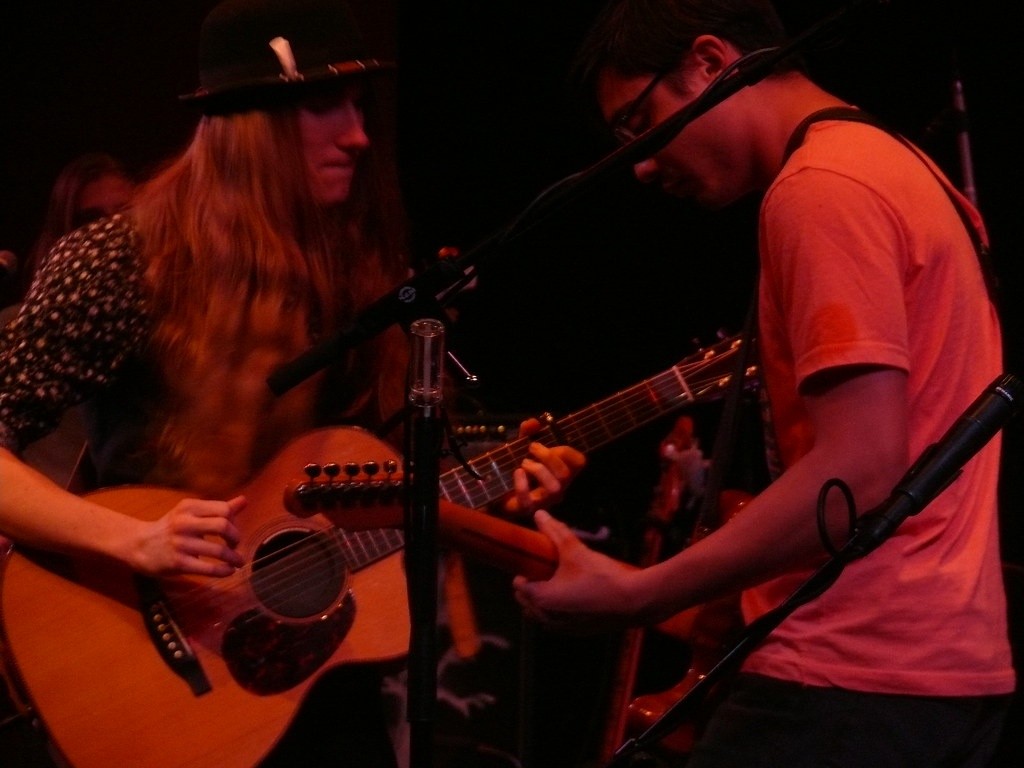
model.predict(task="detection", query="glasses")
[607,49,690,141]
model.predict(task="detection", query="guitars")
[271,439,754,655]
[0,325,772,768]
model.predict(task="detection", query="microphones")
[0,250,17,276]
[952,78,976,209]
[849,368,1024,558]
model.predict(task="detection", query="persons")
[27,152,133,272]
[0,0,587,766]
[511,1,1018,768]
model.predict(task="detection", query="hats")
[175,1,389,106]
